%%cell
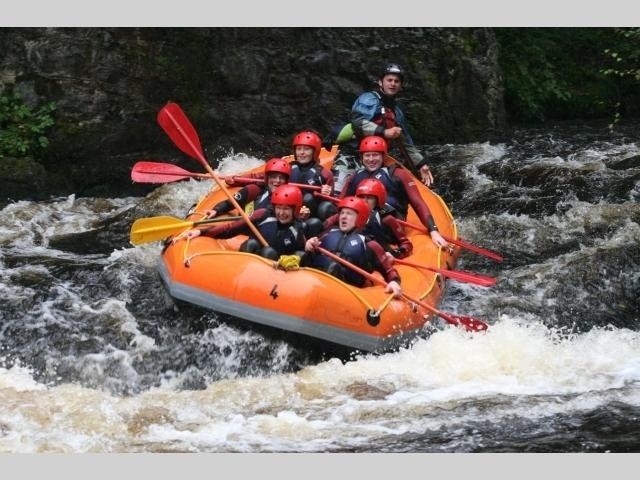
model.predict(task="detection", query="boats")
[158,140,467,360]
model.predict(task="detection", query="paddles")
[158,102,269,247]
[315,245,487,333]
[131,216,246,245]
[394,260,496,287]
[132,162,325,191]
[313,191,504,262]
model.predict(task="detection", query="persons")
[299,196,402,298]
[202,158,321,239]
[322,178,412,265]
[330,63,433,197]
[183,185,306,260]
[225,131,338,222]
[338,136,454,252]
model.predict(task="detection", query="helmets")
[355,179,386,208]
[380,63,404,83]
[271,185,303,219]
[293,132,320,161]
[337,196,370,229]
[359,135,387,154]
[265,158,291,182]
